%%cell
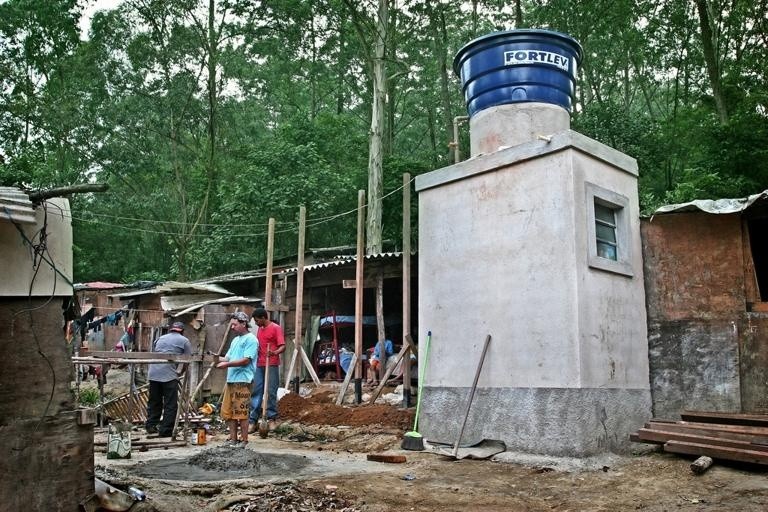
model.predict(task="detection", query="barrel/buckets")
[452,29,584,118]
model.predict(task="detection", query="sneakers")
[268,418,276,432]
[232,440,247,449]
[248,424,257,434]
[218,439,236,447]
[147,426,158,432]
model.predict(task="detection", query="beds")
[314,310,402,382]
[362,346,418,382]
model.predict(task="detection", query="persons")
[247,308,286,433]
[370,335,393,387]
[216,311,259,449]
[146,322,193,436]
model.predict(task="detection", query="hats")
[170,322,184,331]
[233,312,252,328]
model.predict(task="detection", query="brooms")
[401,332,431,451]
